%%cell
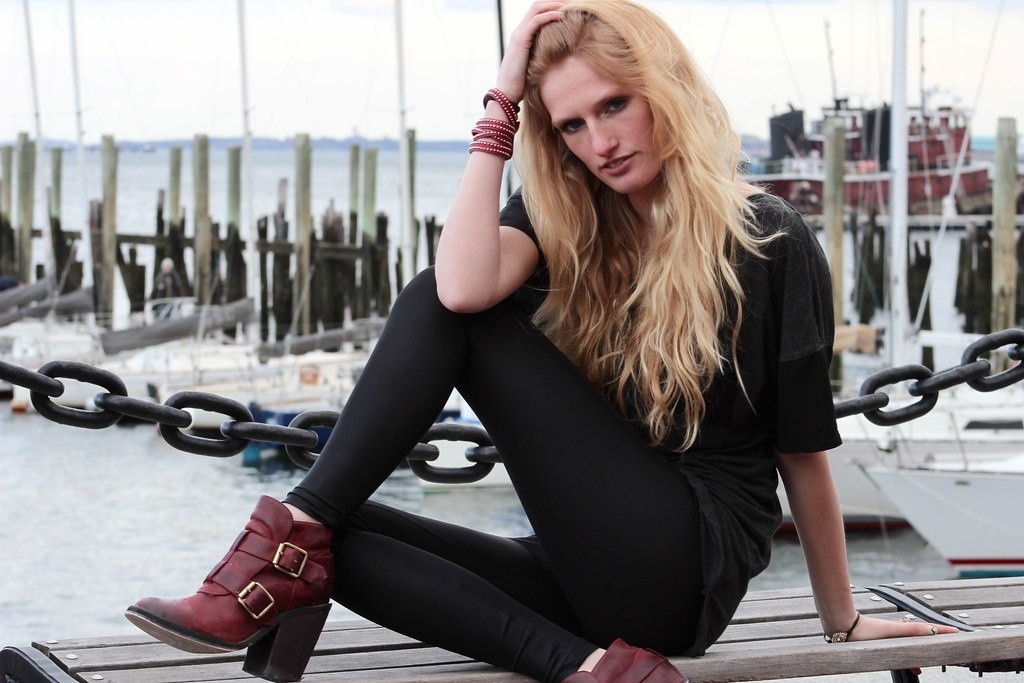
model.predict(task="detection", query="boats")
[2,0,1023,579]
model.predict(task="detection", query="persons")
[122,0,965,683]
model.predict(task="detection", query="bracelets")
[469,88,523,163]
[822,611,863,645]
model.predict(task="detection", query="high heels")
[561,637,691,683]
[125,495,336,682]
